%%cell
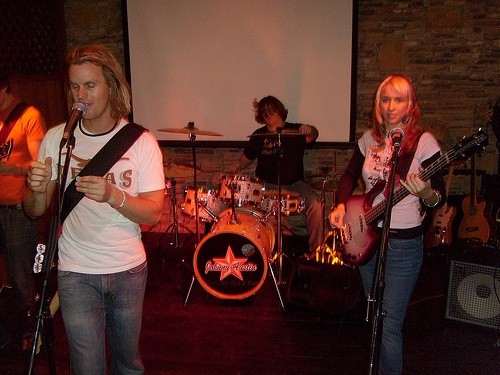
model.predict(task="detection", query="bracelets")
[422,190,441,207]
[112,184,126,209]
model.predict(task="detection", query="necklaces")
[79,119,118,137]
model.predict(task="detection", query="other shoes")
[22,331,35,352]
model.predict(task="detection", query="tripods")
[308,177,338,264]
[271,135,294,288]
[155,179,196,250]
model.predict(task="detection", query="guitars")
[458,128,496,249]
[337,127,489,267]
[0,138,13,160]
[423,165,458,251]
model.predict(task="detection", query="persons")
[0,71,47,357]
[24,43,166,375]
[224,95,324,253]
[328,73,450,375]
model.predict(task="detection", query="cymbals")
[163,161,203,178]
[311,172,344,177]
[247,132,311,136]
[310,180,340,192]
[155,128,223,136]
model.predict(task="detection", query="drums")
[255,190,306,217]
[217,174,265,206]
[180,185,229,223]
[192,207,275,300]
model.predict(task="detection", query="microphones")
[60,102,86,151]
[389,128,405,150]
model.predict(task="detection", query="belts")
[0,205,24,209]
[377,225,424,239]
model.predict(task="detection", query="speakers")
[446,249,500,330]
[286,259,366,315]
